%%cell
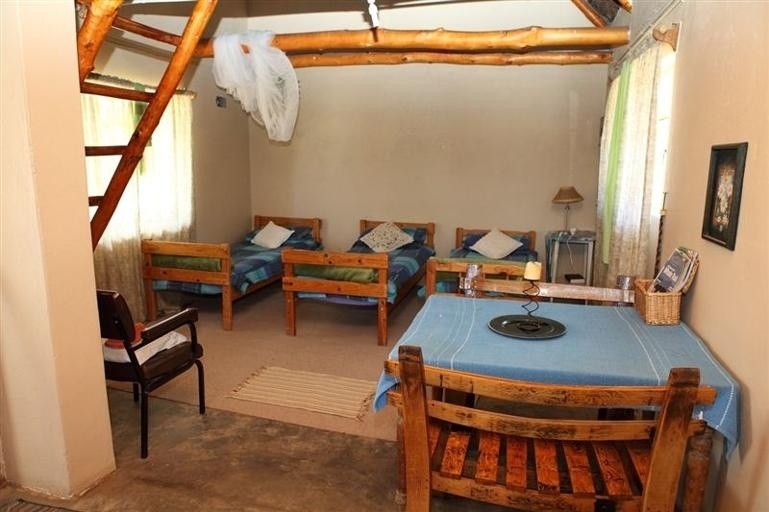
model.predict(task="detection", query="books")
[650,245,699,297]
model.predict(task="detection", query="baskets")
[635,278,683,326]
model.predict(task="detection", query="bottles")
[464,264,485,298]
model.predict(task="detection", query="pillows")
[467,229,524,259]
[249,220,295,249]
[242,226,315,245]
[360,221,414,251]
[462,234,530,256]
[350,228,427,250]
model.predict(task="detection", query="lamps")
[549,185,584,233]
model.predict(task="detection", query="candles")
[523,260,541,279]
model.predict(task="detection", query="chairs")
[95,288,206,460]
[459,265,638,421]
[383,345,719,510]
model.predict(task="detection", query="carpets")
[223,364,378,421]
[107,289,422,444]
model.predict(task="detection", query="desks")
[549,230,594,304]
[372,294,740,511]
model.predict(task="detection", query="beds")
[283,218,436,343]
[140,214,321,329]
[424,225,545,300]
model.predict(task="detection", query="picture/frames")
[699,140,747,251]
